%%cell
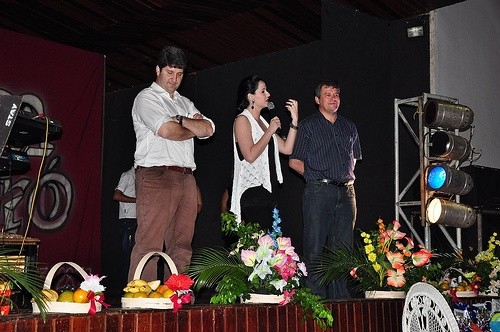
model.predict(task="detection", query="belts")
[307,178,347,188]
[136,164,193,174]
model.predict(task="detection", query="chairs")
[401,281,460,332]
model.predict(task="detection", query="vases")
[239,290,290,304]
[364,290,407,300]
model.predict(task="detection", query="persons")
[221,183,232,250]
[230,78,298,228]
[289,80,362,297]
[115,167,138,295]
[127,47,216,283]
[195,185,203,213]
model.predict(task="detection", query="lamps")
[421,97,481,229]
[406,16,423,37]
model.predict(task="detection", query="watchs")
[176,115,180,123]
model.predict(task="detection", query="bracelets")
[290,123,298,129]
[179,116,183,125]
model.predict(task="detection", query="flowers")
[163,273,194,290]
[308,216,500,307]
[78,273,107,292]
[186,207,334,331]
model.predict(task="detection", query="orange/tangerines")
[441,283,473,291]
[59,289,89,302]
[125,285,174,298]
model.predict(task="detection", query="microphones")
[267,101,280,133]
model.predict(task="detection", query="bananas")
[31,289,59,301]
[123,279,151,295]
